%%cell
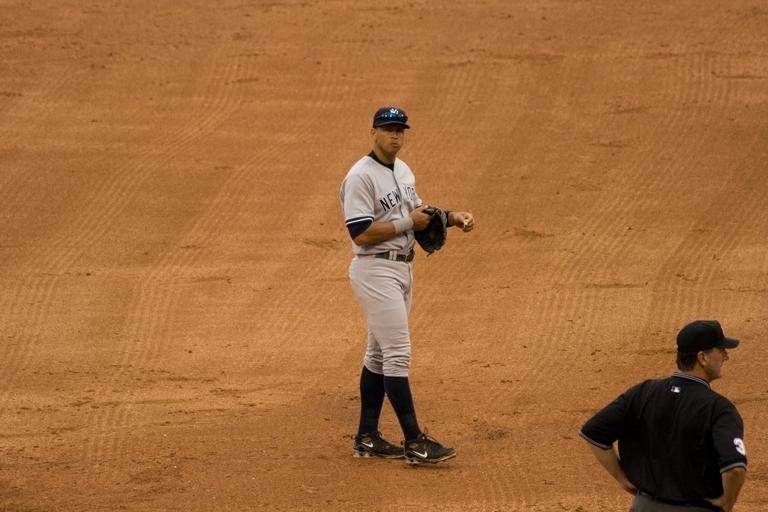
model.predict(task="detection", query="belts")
[634,488,721,510]
[374,248,416,263]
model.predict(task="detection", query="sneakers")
[400,435,457,466]
[352,430,405,459]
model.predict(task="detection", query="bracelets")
[448,211,457,226]
[391,218,415,234]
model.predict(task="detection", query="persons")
[340,108,475,463]
[578,320,748,510]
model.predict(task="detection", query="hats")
[677,319,740,353]
[373,106,411,129]
